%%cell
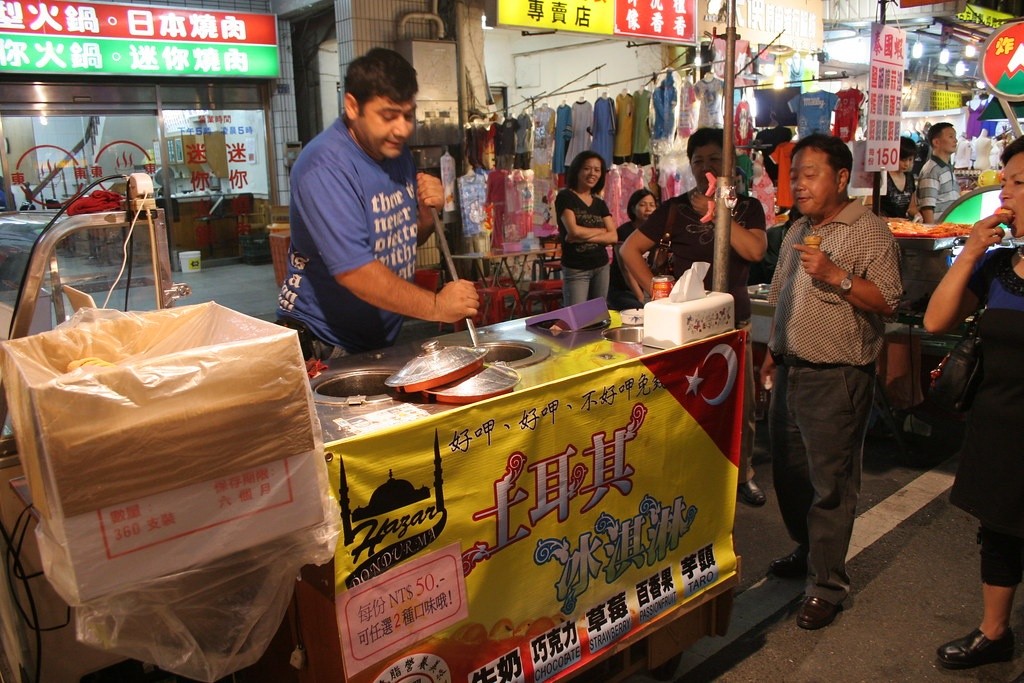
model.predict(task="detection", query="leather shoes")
[769,547,809,578]
[738,480,766,504]
[936,626,1016,669]
[796,595,839,628]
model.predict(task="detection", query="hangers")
[460,64,718,181]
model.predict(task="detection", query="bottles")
[759,283,768,294]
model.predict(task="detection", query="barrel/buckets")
[416,269,439,293]
[178,251,201,273]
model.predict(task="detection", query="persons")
[618,126,768,507]
[922,135,1024,668]
[880,136,923,224]
[271,47,479,365]
[615,189,656,310]
[904,124,920,142]
[955,129,992,170]
[916,122,963,223]
[758,128,904,631]
[554,150,619,308]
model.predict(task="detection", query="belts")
[768,349,850,370]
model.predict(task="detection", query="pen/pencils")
[828,252,832,258]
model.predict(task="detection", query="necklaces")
[1018,244,1024,260]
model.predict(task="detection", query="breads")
[993,207,1015,214]
[66,357,120,372]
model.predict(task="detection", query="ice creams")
[802,232,823,249]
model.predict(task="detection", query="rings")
[993,229,996,235]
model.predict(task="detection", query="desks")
[452,248,559,325]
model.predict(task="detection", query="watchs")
[839,273,853,295]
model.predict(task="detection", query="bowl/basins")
[501,243,523,252]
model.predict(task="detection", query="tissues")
[641,262,735,350]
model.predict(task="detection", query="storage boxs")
[0,300,326,603]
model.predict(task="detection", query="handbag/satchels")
[929,246,1006,413]
[648,197,677,276]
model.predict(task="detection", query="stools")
[428,255,563,333]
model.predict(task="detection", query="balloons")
[978,170,1000,187]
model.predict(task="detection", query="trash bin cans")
[268,221,291,287]
